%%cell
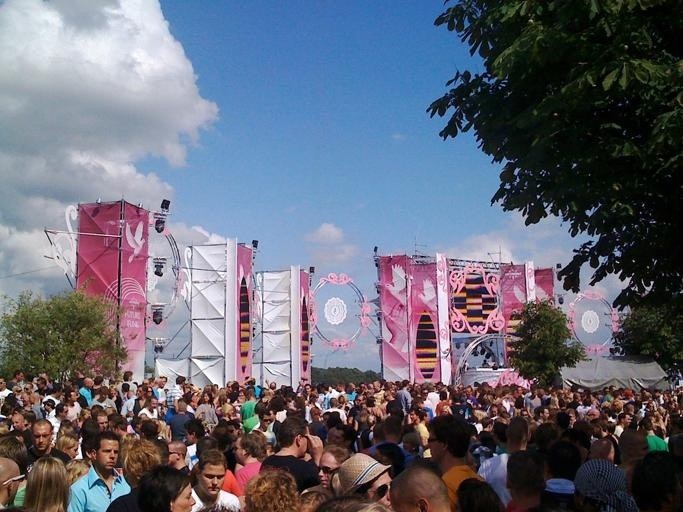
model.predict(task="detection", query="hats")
[43,399,56,409]
[338,453,392,496]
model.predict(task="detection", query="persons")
[0,370,683,512]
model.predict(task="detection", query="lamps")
[308,266,318,282]
[373,244,384,350]
[251,238,259,260]
[551,262,567,307]
[144,197,174,355]
[136,202,143,210]
[94,198,101,205]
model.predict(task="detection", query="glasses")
[317,466,339,474]
[367,481,392,498]
